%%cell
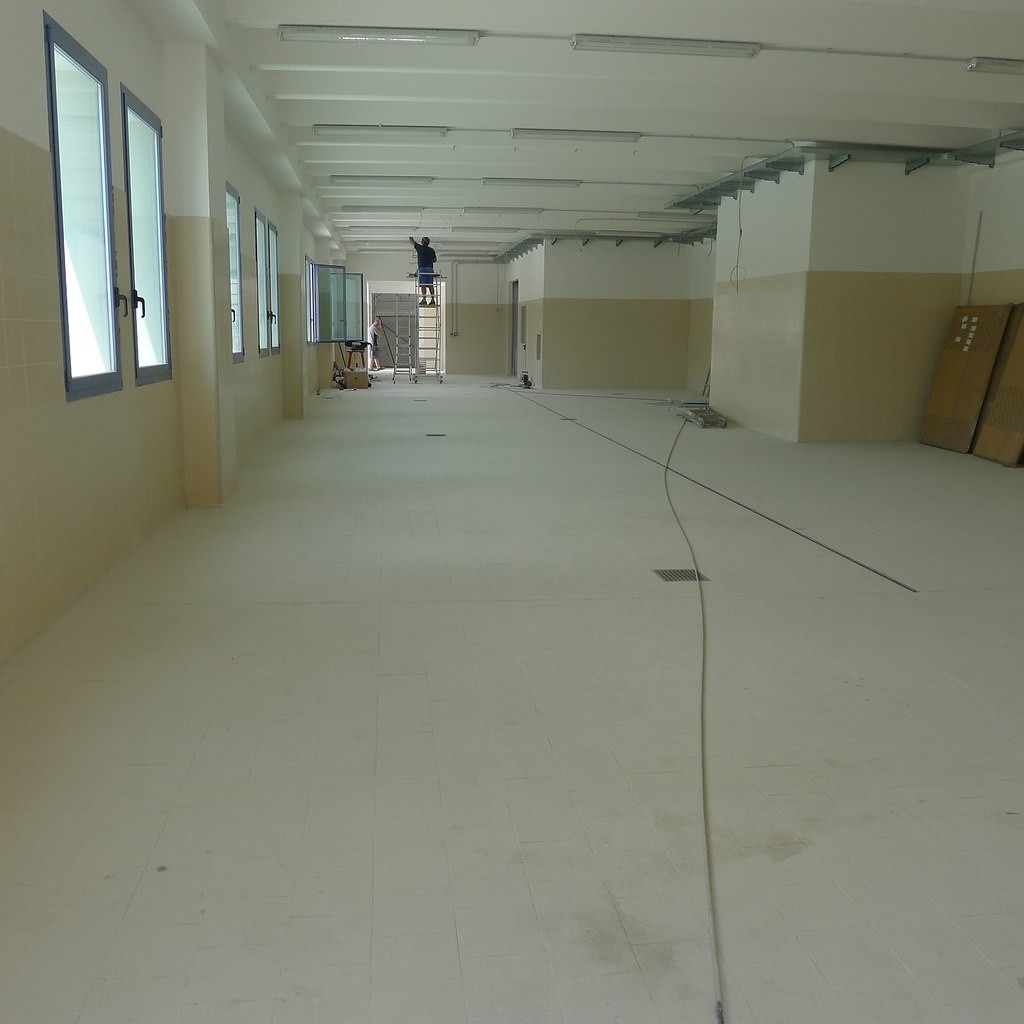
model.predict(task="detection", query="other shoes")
[368,367,378,371]
[419,299,427,305]
[429,301,435,305]
[377,367,385,370]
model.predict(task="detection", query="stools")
[346,349,365,368]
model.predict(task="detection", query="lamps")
[440,248,498,256]
[277,24,481,48]
[312,123,447,138]
[349,225,418,233]
[481,178,581,188]
[341,205,424,214]
[963,55,1024,75]
[331,175,436,188]
[442,240,501,247]
[571,34,760,59]
[509,128,641,143]
[450,226,519,234]
[359,248,411,254]
[638,211,712,220]
[462,206,544,216]
[355,239,411,245]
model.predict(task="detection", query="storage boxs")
[342,368,368,389]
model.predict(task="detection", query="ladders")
[393,294,411,384]
[415,269,444,383]
[669,405,727,428]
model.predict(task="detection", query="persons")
[368,318,385,371]
[409,237,437,305]
